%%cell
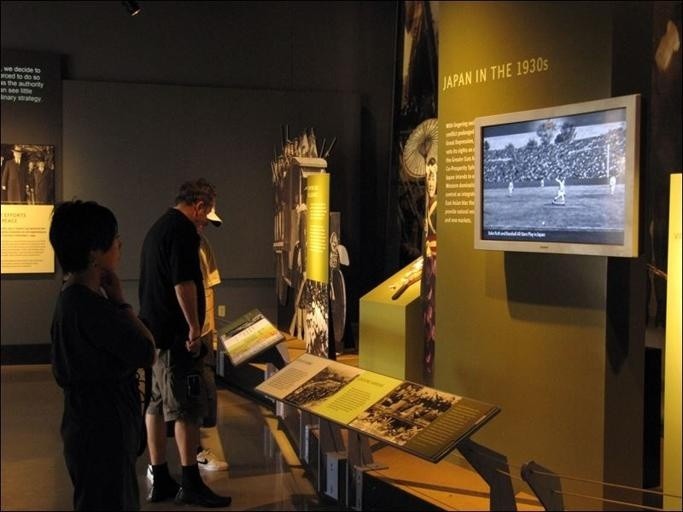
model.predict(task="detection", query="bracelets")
[115,303,132,319]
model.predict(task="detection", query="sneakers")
[200,426,223,460]
[197,450,228,472]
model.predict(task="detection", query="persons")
[552,176,566,204]
[483,133,625,181]
[0,145,53,205]
[139,177,231,508]
[608,176,616,195]
[190,203,227,471]
[508,180,513,197]
[47,201,158,510]
[540,178,544,188]
[424,158,438,385]
[292,372,456,445]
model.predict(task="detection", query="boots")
[147,463,180,502]
[176,467,232,506]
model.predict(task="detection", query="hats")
[206,207,222,228]
[11,146,23,153]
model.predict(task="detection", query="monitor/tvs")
[474,94,640,257]
